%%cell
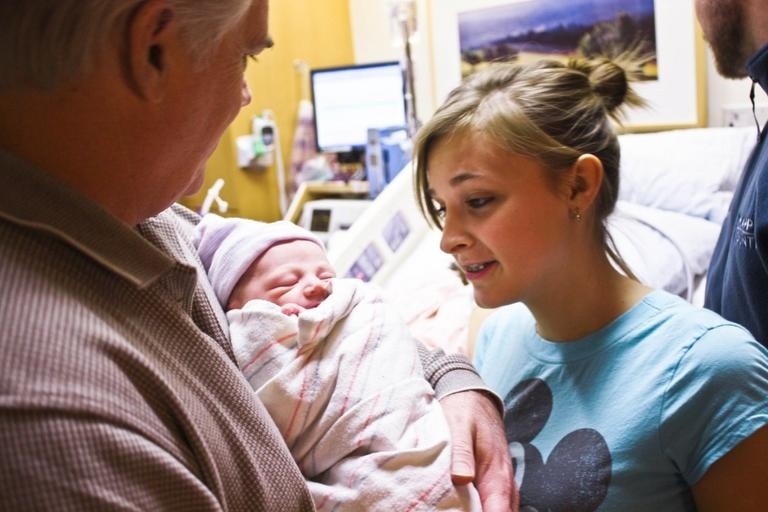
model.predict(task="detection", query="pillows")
[604,202,724,296]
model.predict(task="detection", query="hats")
[192,213,331,310]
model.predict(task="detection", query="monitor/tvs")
[310,59,407,162]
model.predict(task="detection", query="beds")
[328,125,763,348]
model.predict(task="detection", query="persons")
[191,210,483,509]
[1,1,525,510]
[407,26,768,512]
[692,0,768,343]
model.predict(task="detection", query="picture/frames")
[423,0,709,132]
